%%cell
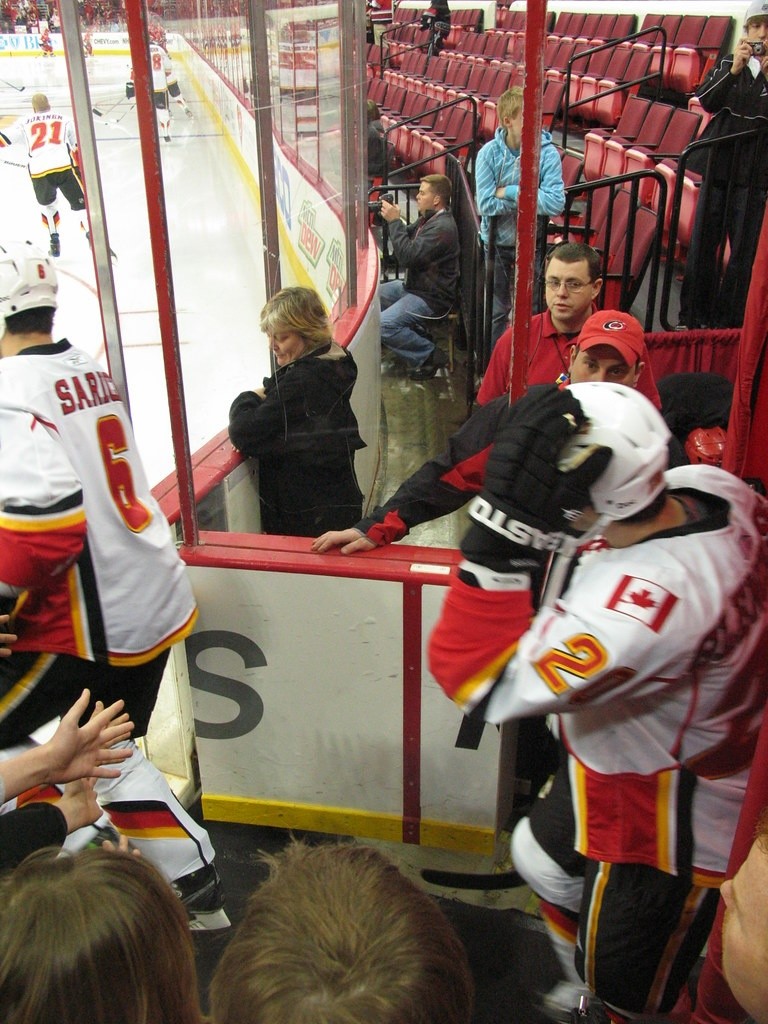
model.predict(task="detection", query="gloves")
[460,385,616,573]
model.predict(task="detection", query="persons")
[149,11,193,142]
[380,174,460,379]
[678,0,768,331]
[424,382,768,1024]
[0,689,202,1024]
[477,242,662,411]
[0,231,232,937]
[423,0,451,56]
[0,93,119,265]
[367,100,386,176]
[208,840,475,1024]
[475,86,566,350]
[0,0,127,34]
[39,28,56,57]
[228,287,368,538]
[311,310,646,872]
[83,28,94,56]
[366,0,393,48]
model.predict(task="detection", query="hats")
[743,0,768,25]
[575,309,645,371]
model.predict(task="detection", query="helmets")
[0,241,59,318]
[557,381,672,523]
[682,427,728,467]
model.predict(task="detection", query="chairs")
[360,1,767,316]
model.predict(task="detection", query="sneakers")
[108,250,118,265]
[184,108,193,117]
[168,862,231,931]
[163,134,172,144]
[49,240,60,256]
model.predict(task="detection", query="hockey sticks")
[111,102,136,123]
[419,864,526,891]
[36,50,43,58]
[92,95,126,117]
[0,158,27,168]
[0,78,26,92]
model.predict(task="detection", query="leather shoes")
[412,347,449,379]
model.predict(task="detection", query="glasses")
[541,276,595,293]
